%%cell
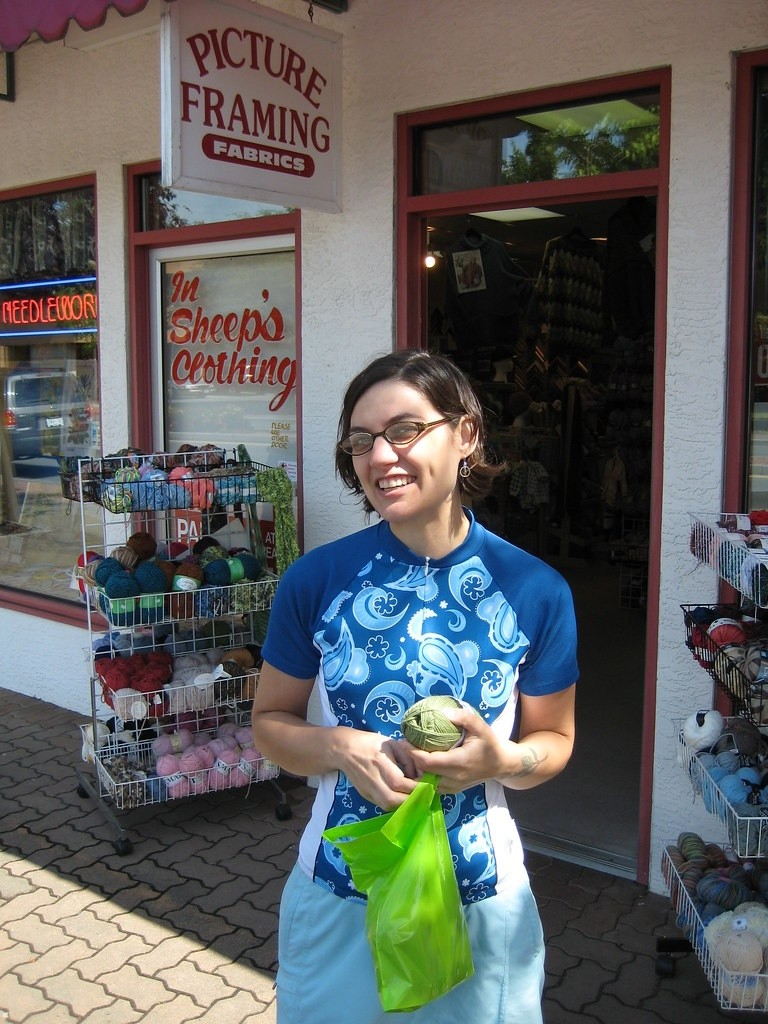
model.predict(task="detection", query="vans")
[0,368,92,466]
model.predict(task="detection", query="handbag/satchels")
[321,772,476,1013]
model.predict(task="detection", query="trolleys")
[68,445,294,855]
[658,511,768,1011]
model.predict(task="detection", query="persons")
[252,350,582,1024]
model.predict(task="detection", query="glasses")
[336,413,464,455]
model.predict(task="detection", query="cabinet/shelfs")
[653,510,768,1024]
[57,448,293,857]
